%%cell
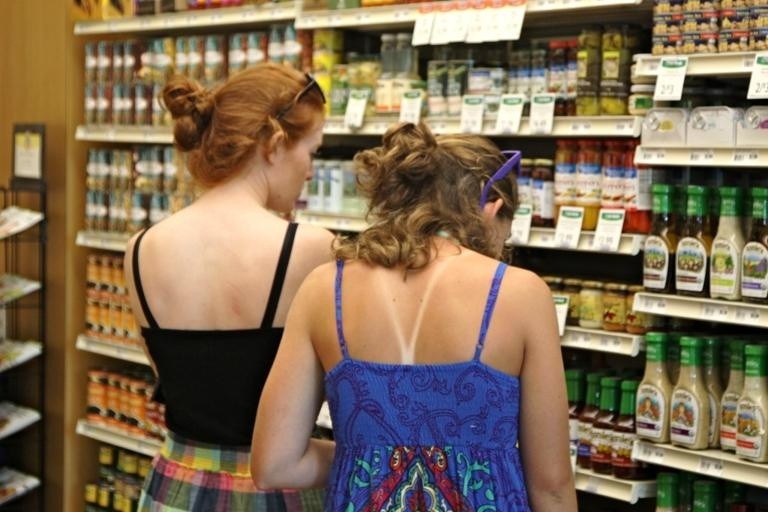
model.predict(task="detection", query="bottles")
[636,182,768,512]
[552,139,668,233]
[380,35,420,79]
[563,363,650,481]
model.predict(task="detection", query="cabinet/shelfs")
[62,1,768,512]
[0,122,48,512]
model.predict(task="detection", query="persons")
[249,119,580,512]
[123,61,342,512]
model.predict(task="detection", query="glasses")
[274,71,327,122]
[478,150,522,211]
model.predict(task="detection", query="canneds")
[85,368,151,436]
[562,279,582,327]
[604,284,626,333]
[427,23,660,117]
[626,286,650,334]
[83,254,145,344]
[84,445,154,511]
[517,158,559,226]
[578,280,606,328]
[539,277,561,296]
